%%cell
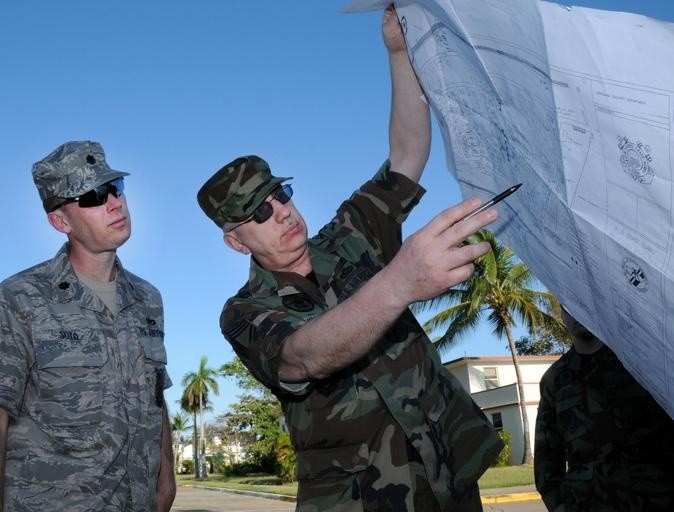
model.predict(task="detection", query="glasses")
[50,176,124,212]
[228,184,293,230]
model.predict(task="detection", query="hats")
[197,155,293,228]
[32,141,130,199]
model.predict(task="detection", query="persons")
[533,302,674,512]
[196,0,506,511]
[2,139,175,511]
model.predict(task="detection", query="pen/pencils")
[453,183,524,227]
